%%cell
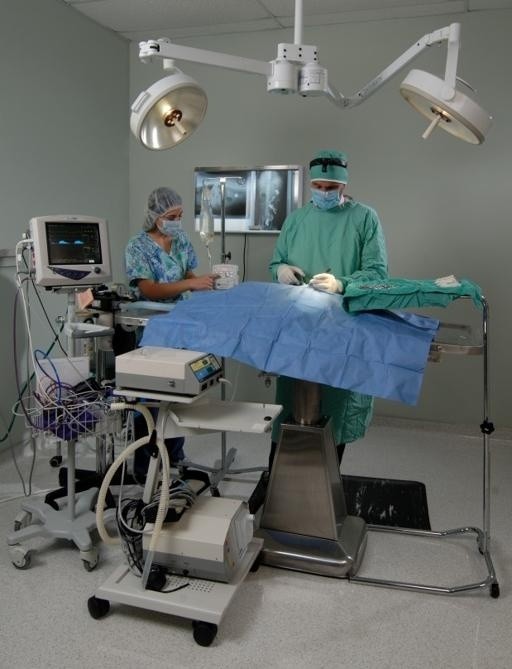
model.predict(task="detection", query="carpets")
[247,467,434,537]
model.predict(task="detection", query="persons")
[125,186,220,487]
[268,150,388,471]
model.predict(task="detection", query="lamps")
[130,0,494,151]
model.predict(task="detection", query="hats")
[309,149,348,185]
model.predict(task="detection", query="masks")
[159,220,183,238]
[309,187,340,211]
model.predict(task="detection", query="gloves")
[277,264,305,286]
[309,273,343,294]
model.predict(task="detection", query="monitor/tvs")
[30,213,113,287]
